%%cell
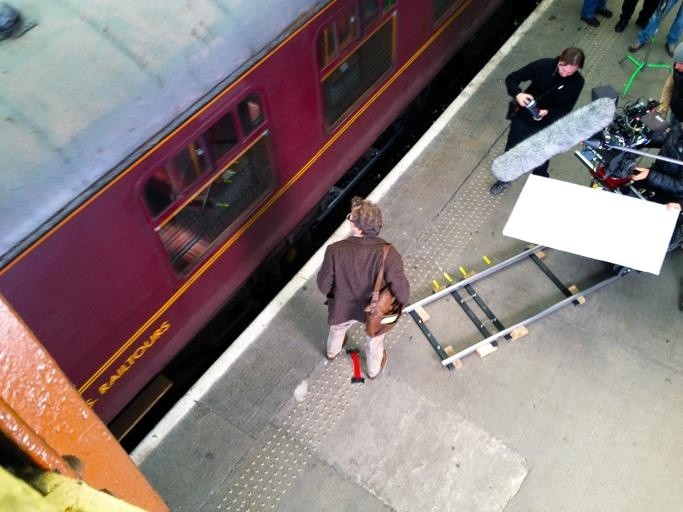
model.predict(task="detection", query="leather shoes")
[326,334,347,359]
[367,349,387,380]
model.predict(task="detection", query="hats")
[673,43,683,64]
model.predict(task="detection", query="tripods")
[619,0,672,99]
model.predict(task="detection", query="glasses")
[346,213,354,224]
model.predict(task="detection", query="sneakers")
[491,180,511,194]
[581,7,648,32]
[629,39,642,52]
[665,41,676,56]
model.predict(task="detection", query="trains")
[0,0,506,428]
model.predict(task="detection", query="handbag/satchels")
[366,286,403,338]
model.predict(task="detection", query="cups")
[526,100,542,121]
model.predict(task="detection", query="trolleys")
[573,99,683,278]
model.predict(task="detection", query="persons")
[615,0,660,32]
[316,197,410,380]
[628,0,683,58]
[580,0,612,27]
[489,47,585,196]
[631,121,683,253]
[658,42,683,125]
